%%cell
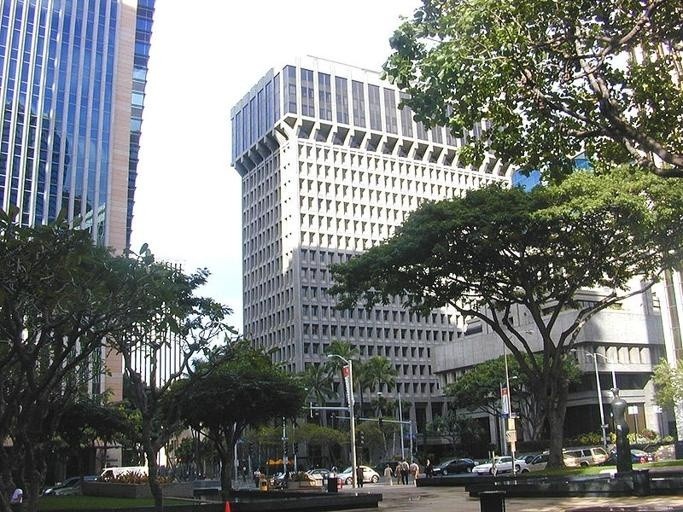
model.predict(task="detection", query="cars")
[42,476,101,498]
[337,465,380,484]
[277,468,331,480]
[428,445,655,478]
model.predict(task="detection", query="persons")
[357,465,364,487]
[424,458,435,478]
[410,460,419,484]
[9,486,24,505]
[400,458,409,485]
[253,468,261,488]
[395,462,402,483]
[384,463,394,484]
[490,458,500,478]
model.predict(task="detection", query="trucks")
[95,466,149,482]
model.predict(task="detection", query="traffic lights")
[378,417,384,430]
[313,403,319,418]
[357,430,364,445]
[356,414,361,426]
[331,415,337,429]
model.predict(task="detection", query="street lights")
[502,330,533,474]
[326,354,358,487]
[570,348,607,452]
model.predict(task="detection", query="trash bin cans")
[328,478,338,492]
[480,491,506,512]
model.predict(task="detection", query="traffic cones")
[225,500,230,512]
